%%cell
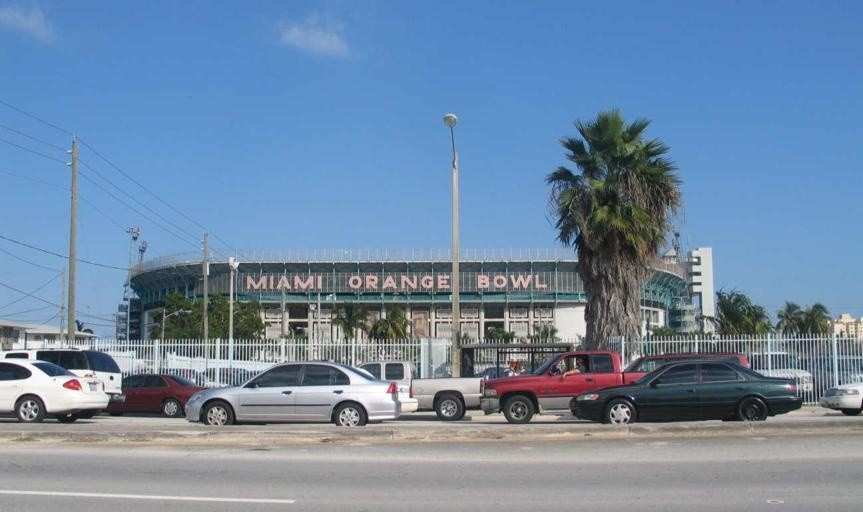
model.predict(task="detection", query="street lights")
[437,108,465,376]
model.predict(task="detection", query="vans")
[625,351,751,378]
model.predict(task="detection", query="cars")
[570,358,805,426]
[751,350,815,396]
[185,361,402,428]
[0,357,111,424]
[104,374,213,417]
[798,354,863,395]
[820,382,863,416]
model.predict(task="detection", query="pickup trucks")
[480,350,652,426]
[355,360,492,421]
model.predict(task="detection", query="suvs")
[4,347,127,418]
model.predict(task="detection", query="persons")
[561,356,589,378]
